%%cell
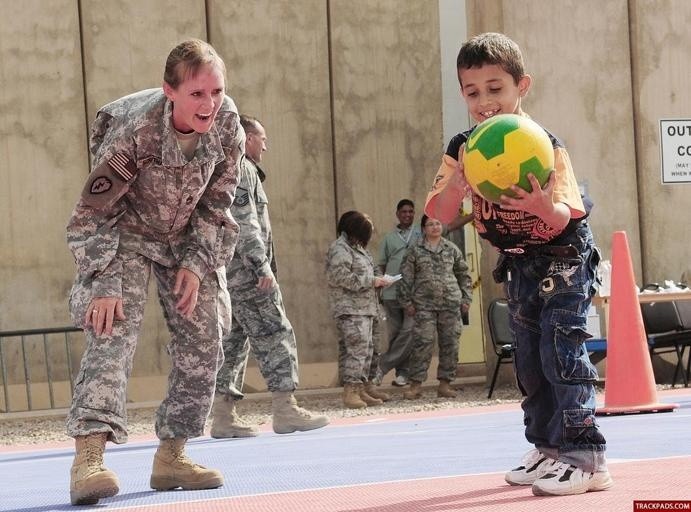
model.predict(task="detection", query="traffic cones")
[592,232,680,416]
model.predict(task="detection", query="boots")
[150,437,224,491]
[272,390,330,434]
[69,431,119,507]
[210,392,256,439]
[342,379,458,408]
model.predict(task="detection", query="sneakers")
[504,447,557,486]
[372,353,385,386]
[533,459,614,496]
[392,375,410,386]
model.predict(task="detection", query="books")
[382,273,403,290]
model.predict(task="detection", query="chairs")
[638,298,691,390]
[669,297,691,390]
[482,295,532,401]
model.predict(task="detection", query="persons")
[64,37,246,506]
[374,198,421,385]
[92,309,98,313]
[209,115,329,440]
[421,31,612,495]
[397,214,473,399]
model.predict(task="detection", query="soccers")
[462,113,554,204]
